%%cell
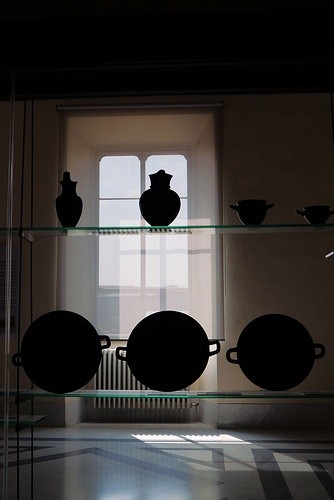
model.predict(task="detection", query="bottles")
[55,171,83,227]
[140,170,180,226]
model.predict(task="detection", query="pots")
[116,312,221,393]
[11,309,110,395]
[226,314,327,392]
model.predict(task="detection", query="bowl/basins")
[295,205,334,224]
[228,199,275,226]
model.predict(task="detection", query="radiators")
[94,349,192,409]
